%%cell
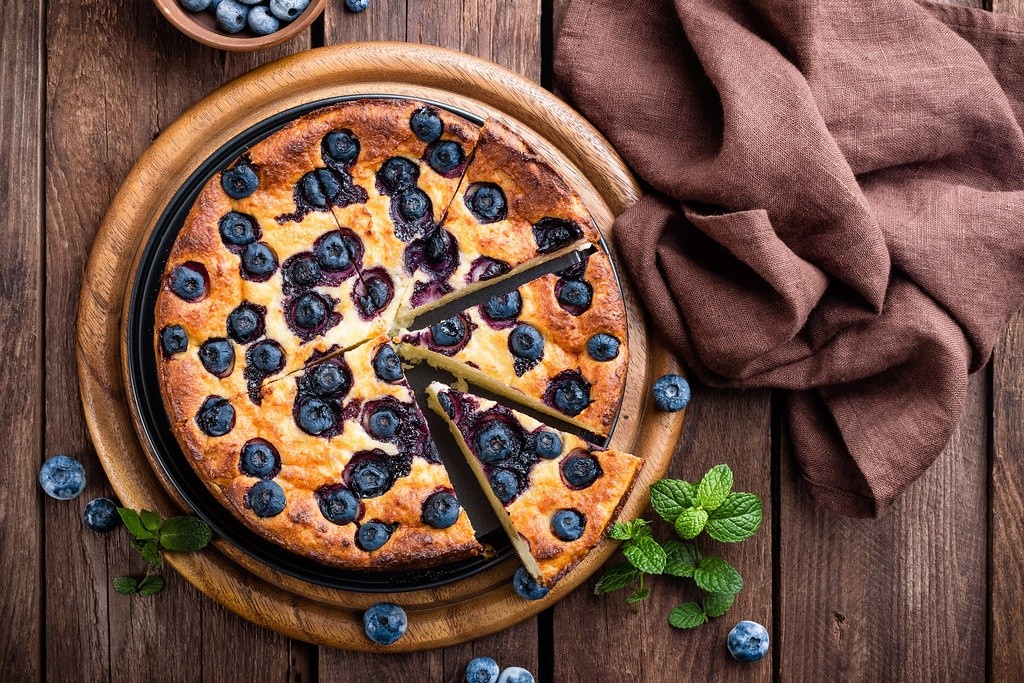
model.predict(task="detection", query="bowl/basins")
[154,0,327,52]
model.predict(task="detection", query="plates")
[72,39,683,649]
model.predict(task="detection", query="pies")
[151,98,648,589]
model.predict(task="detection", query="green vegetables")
[112,507,213,596]
[596,462,764,629]
